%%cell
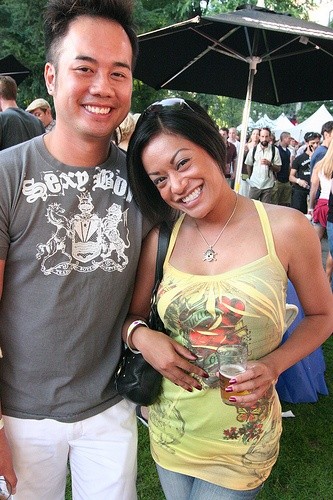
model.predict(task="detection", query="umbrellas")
[0,54,31,86]
[130,4,333,195]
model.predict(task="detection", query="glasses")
[307,133,321,138]
[144,97,195,114]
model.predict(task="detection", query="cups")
[260,157,266,165]
[216,344,247,405]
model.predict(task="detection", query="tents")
[236,117,254,132]
[292,103,333,144]
[273,112,295,141]
[256,114,273,128]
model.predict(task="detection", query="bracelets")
[126,320,148,354]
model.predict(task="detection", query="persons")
[122,98,333,500]
[0,75,45,151]
[25,98,56,134]
[112,112,141,155]
[0,0,172,500]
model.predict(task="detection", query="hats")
[112,113,142,152]
[25,97,50,113]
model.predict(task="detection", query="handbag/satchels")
[113,219,178,406]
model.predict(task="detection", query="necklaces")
[195,191,238,262]
